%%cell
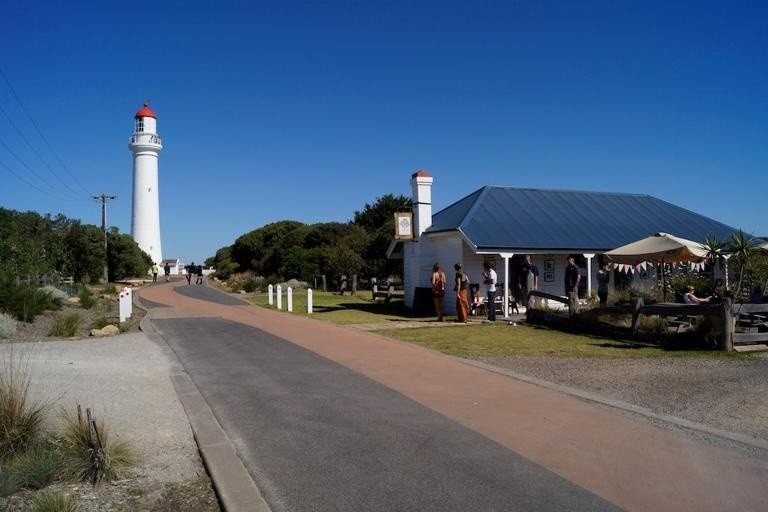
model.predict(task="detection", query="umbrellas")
[600,231,736,303]
[745,238,768,256]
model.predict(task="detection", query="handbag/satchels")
[433,280,442,295]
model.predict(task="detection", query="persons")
[194,265,203,285]
[452,263,470,323]
[430,262,447,322]
[683,284,715,305]
[595,261,611,307]
[517,254,540,315]
[152,263,158,283]
[563,256,581,307]
[185,262,195,287]
[482,259,498,323]
[749,286,767,304]
[164,262,170,281]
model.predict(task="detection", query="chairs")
[503,287,519,315]
[470,283,485,317]
